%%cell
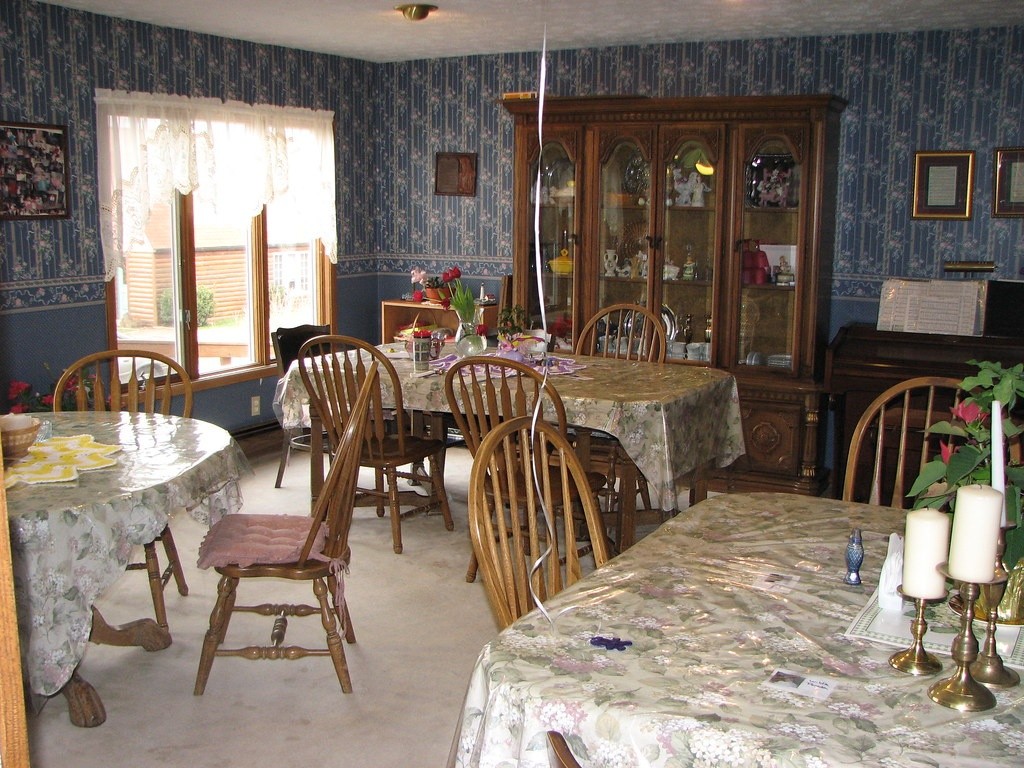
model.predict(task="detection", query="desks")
[271,337,745,553]
[443,493,1024,768]
[0,411,255,727]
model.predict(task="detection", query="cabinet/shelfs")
[381,298,498,345]
[498,95,849,498]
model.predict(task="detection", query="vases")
[499,350,523,363]
[455,323,488,355]
[425,287,456,304]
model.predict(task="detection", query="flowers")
[8,371,112,414]
[497,304,527,351]
[439,267,489,350]
[410,267,444,289]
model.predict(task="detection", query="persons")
[0,129,66,215]
[692,173,708,207]
[779,256,789,275]
[755,166,784,202]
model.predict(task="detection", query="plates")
[622,300,678,341]
[745,352,791,368]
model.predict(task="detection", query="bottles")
[603,249,619,276]
[842,529,864,584]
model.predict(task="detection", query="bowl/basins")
[0,414,42,460]
[547,249,574,275]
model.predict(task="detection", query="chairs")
[468,414,607,630]
[843,377,987,509]
[53,350,192,631]
[194,361,378,696]
[271,304,666,583]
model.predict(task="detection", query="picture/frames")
[0,121,69,220]
[991,146,1024,218]
[911,151,975,221]
[436,153,477,196]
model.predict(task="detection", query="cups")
[776,272,795,285]
[599,333,711,362]
[412,329,431,372]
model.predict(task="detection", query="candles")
[902,505,948,597]
[991,401,1005,526]
[946,484,1003,584]
[481,281,484,299]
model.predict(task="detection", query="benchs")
[869,408,957,462]
